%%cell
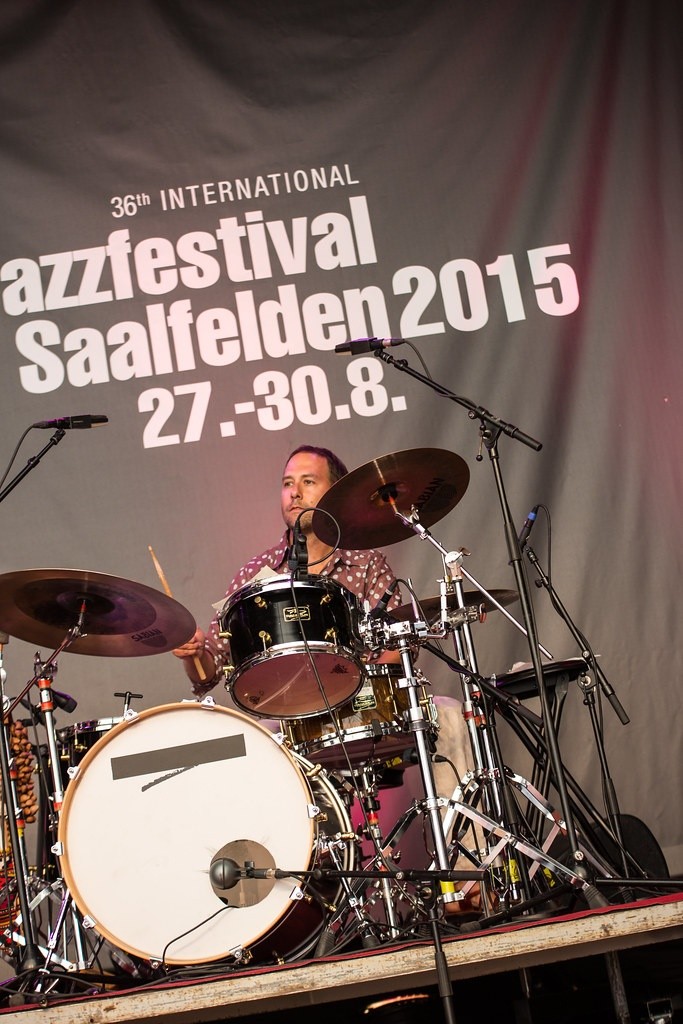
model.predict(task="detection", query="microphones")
[372,580,398,611]
[286,520,302,570]
[508,505,539,566]
[19,680,77,727]
[334,338,405,355]
[32,414,110,430]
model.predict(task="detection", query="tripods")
[397,440,683,932]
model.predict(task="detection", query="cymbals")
[387,589,522,626]
[312,448,470,550]
[0,568,196,658]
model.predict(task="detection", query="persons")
[172,445,514,920]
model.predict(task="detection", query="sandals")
[440,888,502,923]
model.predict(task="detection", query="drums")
[50,698,360,972]
[217,574,366,721]
[282,662,438,770]
